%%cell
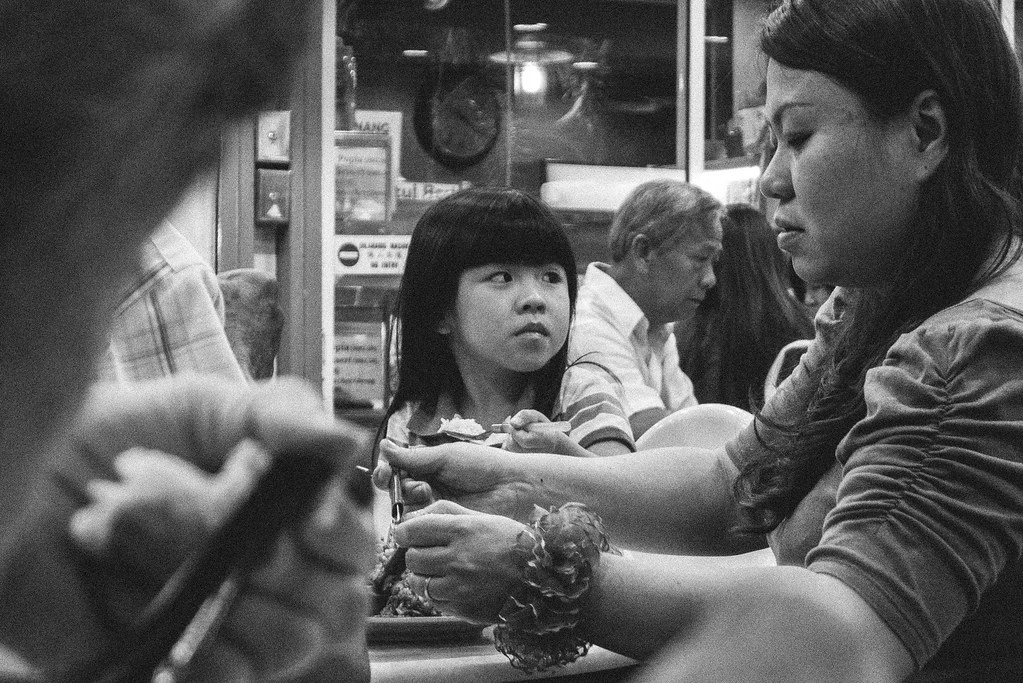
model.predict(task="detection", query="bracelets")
[492,501,609,674]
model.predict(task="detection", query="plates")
[359,616,487,642]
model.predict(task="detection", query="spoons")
[446,422,572,440]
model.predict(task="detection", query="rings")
[425,575,437,603]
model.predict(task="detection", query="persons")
[0,0,1023,683]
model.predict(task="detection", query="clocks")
[414,67,502,174]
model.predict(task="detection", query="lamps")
[488,21,574,97]
